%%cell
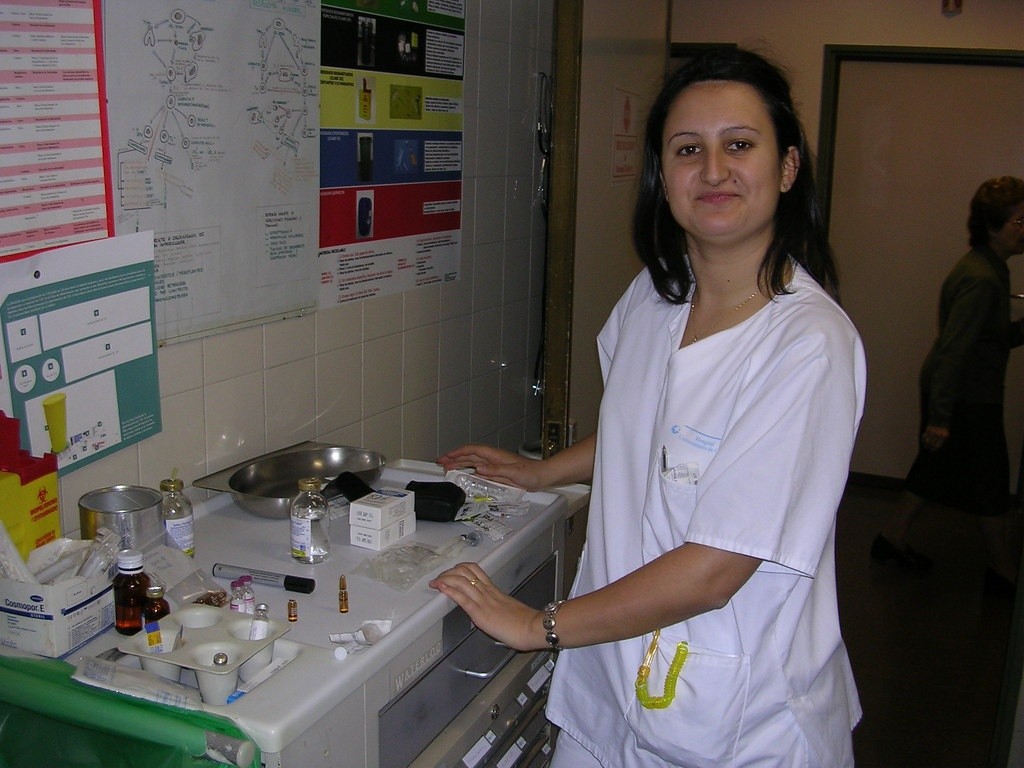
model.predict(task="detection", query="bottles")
[240,575,256,614]
[249,604,270,641]
[290,479,330,564]
[212,652,227,667]
[112,550,149,636]
[338,589,349,613]
[158,466,195,559]
[229,581,247,613]
[339,575,347,590]
[144,586,170,626]
[288,599,297,622]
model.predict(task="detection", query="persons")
[869,177,1024,598]
[428,39,866,768]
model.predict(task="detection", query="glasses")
[1005,218,1024,225]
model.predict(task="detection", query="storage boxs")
[350,512,416,551]
[0,540,116,661]
[349,486,415,531]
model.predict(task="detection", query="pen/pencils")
[662,445,668,470]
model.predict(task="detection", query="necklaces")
[689,282,766,346]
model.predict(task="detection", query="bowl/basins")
[78,484,163,550]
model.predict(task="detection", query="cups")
[358,198,372,237]
[359,89,371,121]
[359,137,372,163]
[43,393,68,453]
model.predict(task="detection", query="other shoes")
[870,532,932,572]
[982,567,1016,599]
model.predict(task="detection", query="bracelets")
[542,599,565,651]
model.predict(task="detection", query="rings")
[470,577,479,585]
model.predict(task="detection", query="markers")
[212,563,315,595]
